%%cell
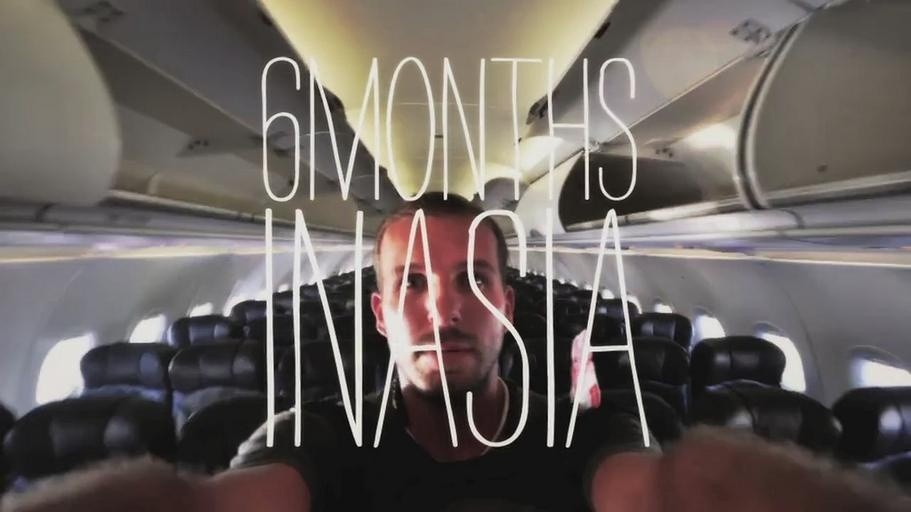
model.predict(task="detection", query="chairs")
[699,376,844,469]
[178,398,292,500]
[632,313,698,344]
[834,388,910,488]
[80,342,168,394]
[616,340,690,413]
[689,336,786,398]
[168,344,269,400]
[0,393,178,495]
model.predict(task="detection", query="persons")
[0,192,911,512]
